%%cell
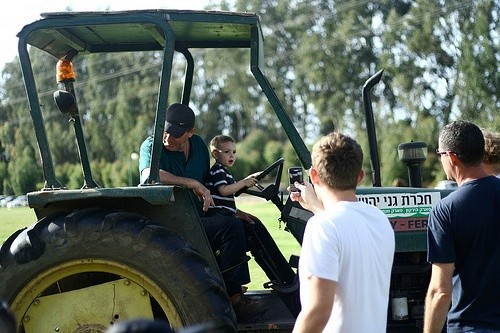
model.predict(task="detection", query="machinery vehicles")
[0,8,460,333]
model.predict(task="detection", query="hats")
[164,103,195,139]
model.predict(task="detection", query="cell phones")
[288,167,304,193]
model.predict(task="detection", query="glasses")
[435,148,464,162]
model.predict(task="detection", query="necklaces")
[186,140,188,160]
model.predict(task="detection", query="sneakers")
[233,297,270,320]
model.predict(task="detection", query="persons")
[287,132,397,333]
[422,121,500,333]
[478,125,500,179]
[392,173,408,187]
[139,103,296,321]
[205,135,270,294]
